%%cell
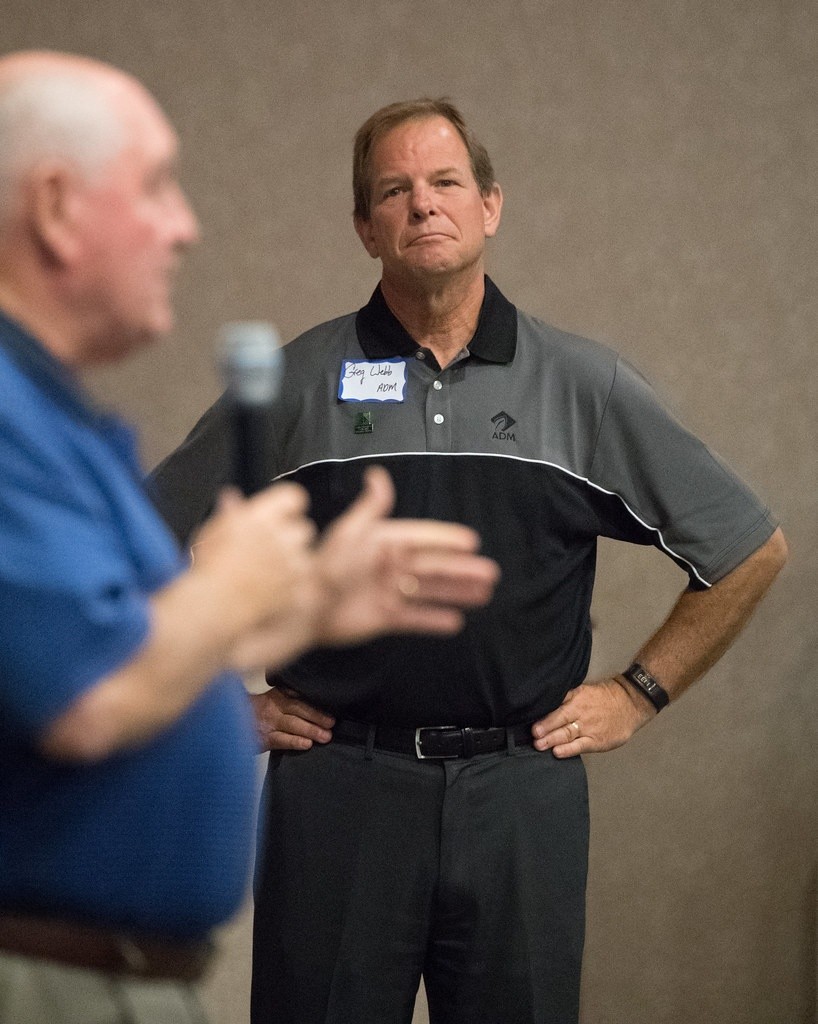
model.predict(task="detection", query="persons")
[0,53,501,1023]
[144,92,787,1023]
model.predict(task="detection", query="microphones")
[220,318,286,499]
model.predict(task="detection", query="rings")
[570,722,583,738]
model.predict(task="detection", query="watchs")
[620,663,670,712]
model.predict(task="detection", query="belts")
[1,908,214,985]
[326,715,549,762]
